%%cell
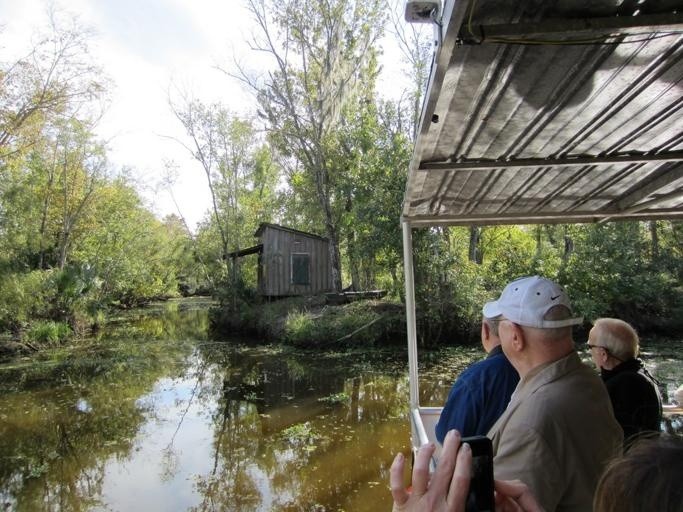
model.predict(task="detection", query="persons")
[435,313,521,447]
[586,318,664,438]
[482,279,624,512]
[390,428,683,512]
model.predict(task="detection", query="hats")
[483,274,575,330]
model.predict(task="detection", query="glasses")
[584,343,606,350]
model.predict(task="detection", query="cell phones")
[452,435,496,512]
